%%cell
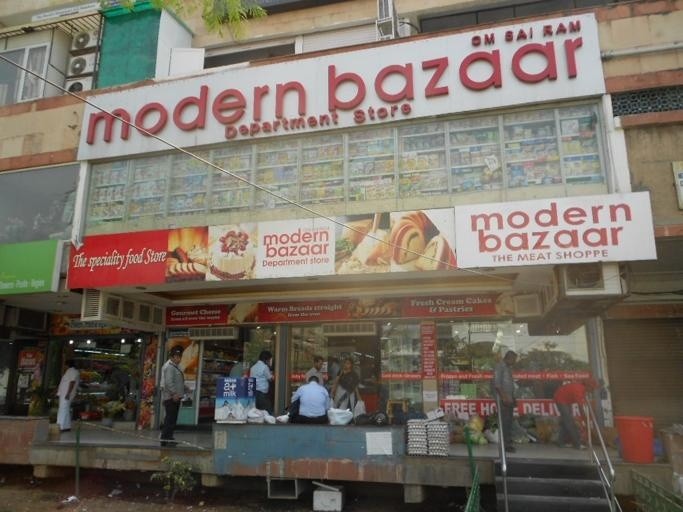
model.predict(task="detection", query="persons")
[228,355,243,376]
[332,358,362,426]
[56,359,81,433]
[305,357,325,386]
[292,375,332,425]
[552,380,598,450]
[160,349,184,446]
[158,345,184,438]
[249,350,275,414]
[493,350,516,452]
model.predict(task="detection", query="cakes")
[165,243,205,281]
[209,231,256,281]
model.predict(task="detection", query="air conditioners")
[63,28,103,95]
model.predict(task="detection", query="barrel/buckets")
[613,415,655,464]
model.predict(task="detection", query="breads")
[355,304,393,317]
[390,210,456,272]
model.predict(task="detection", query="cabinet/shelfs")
[200,342,244,419]
